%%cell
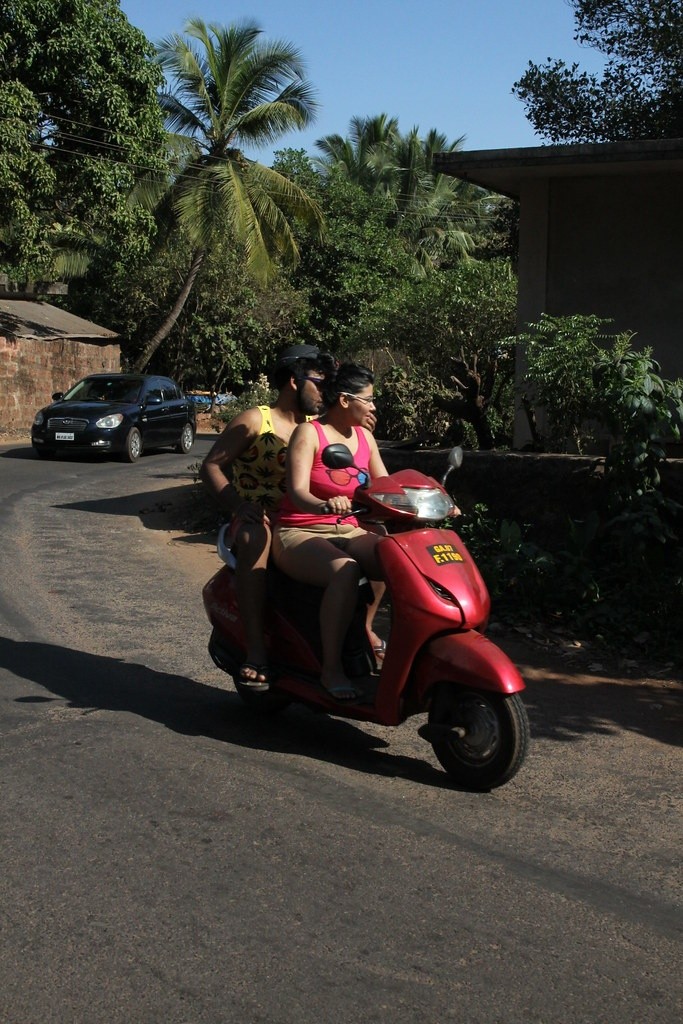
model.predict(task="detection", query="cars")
[30,371,200,461]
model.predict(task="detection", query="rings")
[335,501,340,505]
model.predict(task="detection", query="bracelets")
[235,501,250,512]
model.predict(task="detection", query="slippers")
[237,657,270,691]
[318,673,363,706]
[369,632,389,660]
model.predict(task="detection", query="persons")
[271,361,462,703]
[201,344,388,692]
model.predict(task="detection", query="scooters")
[201,444,533,792]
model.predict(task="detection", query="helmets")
[277,344,334,371]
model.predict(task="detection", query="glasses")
[336,391,377,406]
[302,375,322,390]
